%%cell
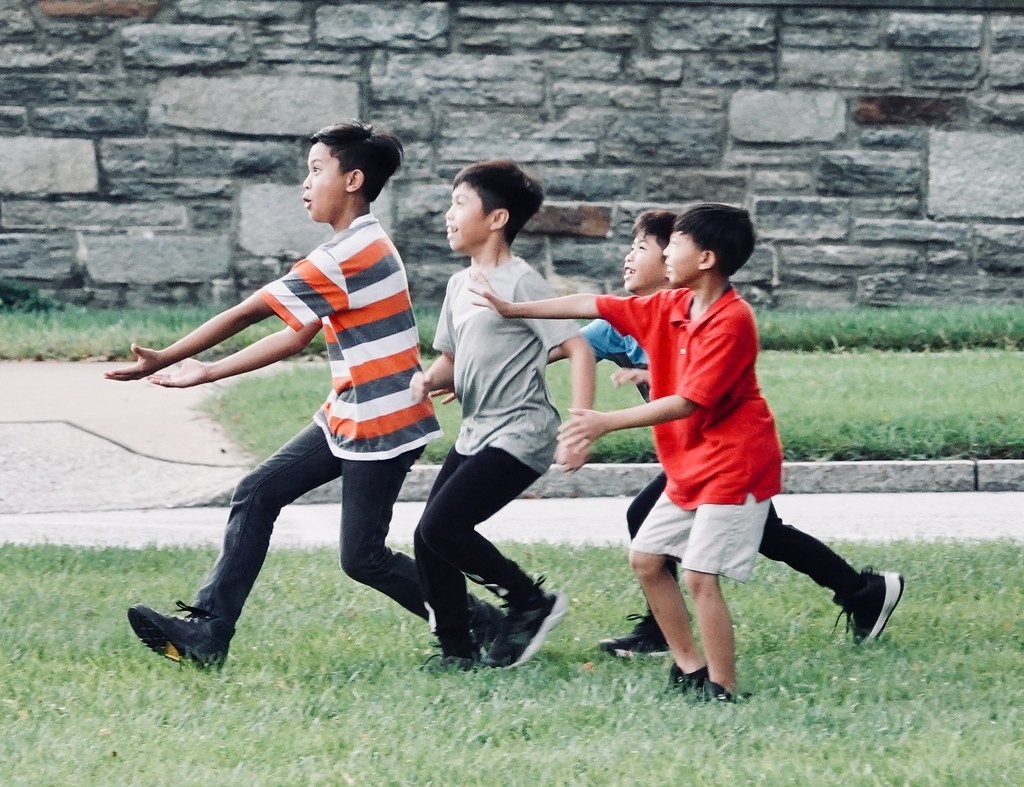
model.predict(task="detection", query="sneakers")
[127,599,235,671]
[693,677,750,703]
[427,591,505,660]
[419,653,480,675]
[663,663,710,695]
[833,566,905,646]
[600,609,672,656]
[480,574,567,669]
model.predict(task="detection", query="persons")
[468,203,781,703]
[429,210,905,656]
[412,158,596,672]
[104,120,504,704]
